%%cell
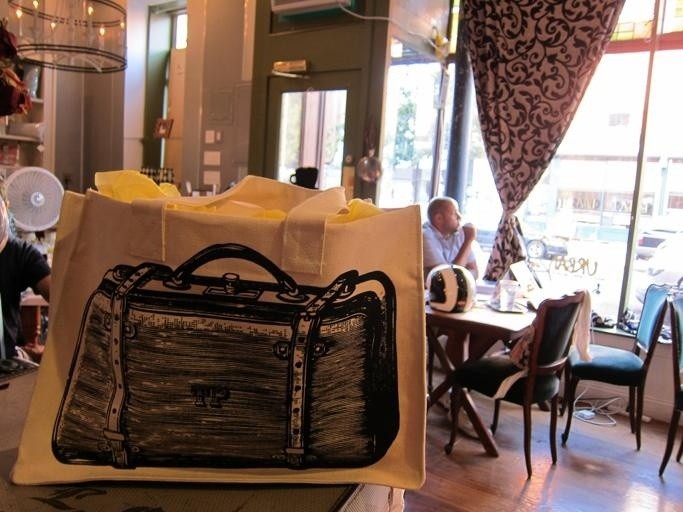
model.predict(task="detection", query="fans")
[3,166,65,256]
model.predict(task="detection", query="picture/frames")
[154,118,175,139]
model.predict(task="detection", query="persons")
[418,196,494,438]
[0,179,52,393]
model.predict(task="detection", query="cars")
[632,224,683,326]
[460,204,570,261]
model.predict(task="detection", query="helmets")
[427,264,475,313]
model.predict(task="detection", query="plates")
[487,300,528,313]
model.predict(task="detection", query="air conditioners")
[271,0,352,15]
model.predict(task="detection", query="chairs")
[659,293,683,476]
[445,289,589,477]
[558,283,671,450]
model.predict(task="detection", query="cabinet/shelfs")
[0,38,56,172]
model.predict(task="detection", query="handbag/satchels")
[50,243,400,469]
[11,176,428,489]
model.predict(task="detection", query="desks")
[426,301,538,457]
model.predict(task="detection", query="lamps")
[0,0,128,73]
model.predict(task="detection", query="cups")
[500,279,519,309]
[289,167,318,188]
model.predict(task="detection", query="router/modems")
[576,408,595,421]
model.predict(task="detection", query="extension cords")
[606,400,652,425]
